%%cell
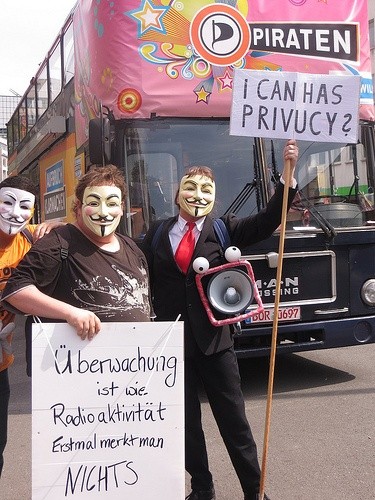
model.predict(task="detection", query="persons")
[0,175,69,477]
[1,162,158,378]
[141,138,298,500]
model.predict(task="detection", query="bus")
[6,0,375,360]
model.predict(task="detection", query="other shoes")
[185,487,216,500]
[244,490,271,500]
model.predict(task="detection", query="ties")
[174,222,196,275]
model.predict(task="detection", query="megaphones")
[207,268,254,335]
